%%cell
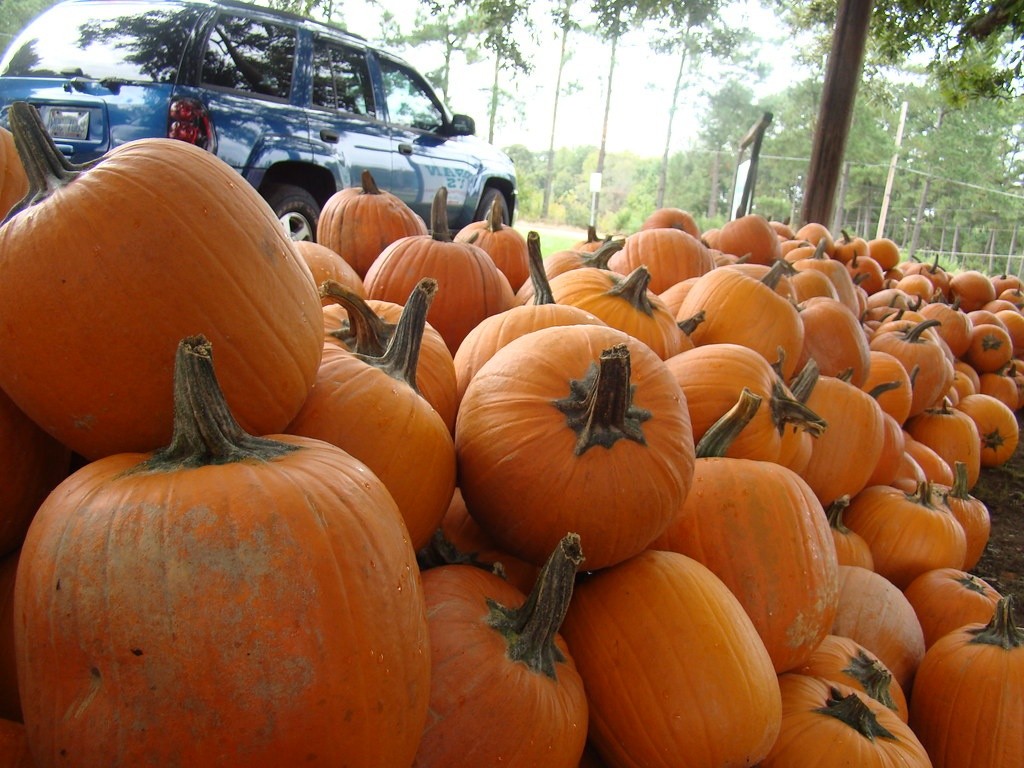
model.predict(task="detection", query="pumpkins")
[0,102,1024,768]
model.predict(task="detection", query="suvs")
[0,0,518,243]
